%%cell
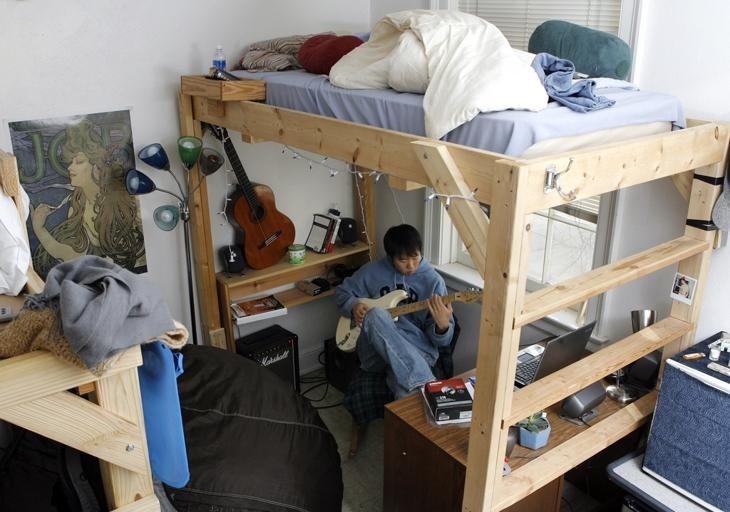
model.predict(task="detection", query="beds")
[0,148,162,512]
[174,31,730,512]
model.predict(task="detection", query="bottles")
[212,46,227,72]
[288,244,306,265]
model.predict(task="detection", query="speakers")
[219,246,246,278]
[560,380,607,419]
[338,218,358,249]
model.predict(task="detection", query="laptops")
[515,319,599,388]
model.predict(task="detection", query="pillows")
[527,18,632,82]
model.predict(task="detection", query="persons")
[29,119,147,272]
[335,224,456,400]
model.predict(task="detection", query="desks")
[383,332,655,512]
[215,239,371,354]
[606,447,709,512]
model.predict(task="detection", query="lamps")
[125,136,225,345]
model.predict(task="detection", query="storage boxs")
[641,331,729,512]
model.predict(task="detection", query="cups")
[708,344,722,362]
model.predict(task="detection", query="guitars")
[210,124,296,270]
[336,287,484,353]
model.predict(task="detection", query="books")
[231,294,288,325]
[305,213,341,254]
